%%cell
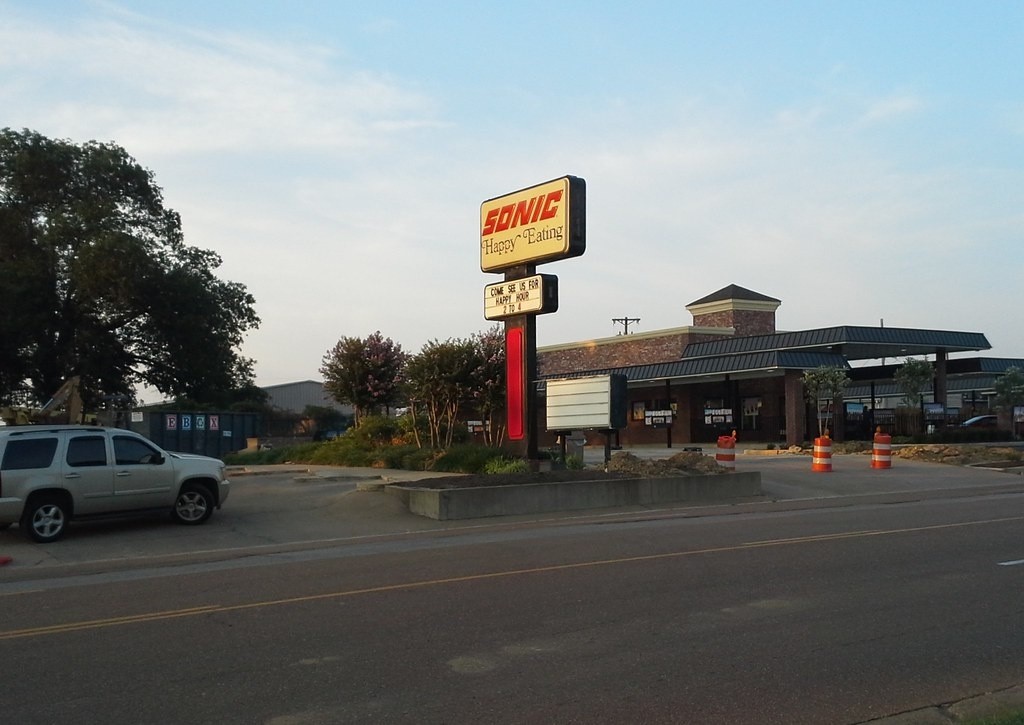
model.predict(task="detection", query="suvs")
[0,424,230,543]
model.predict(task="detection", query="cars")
[947,414,999,432]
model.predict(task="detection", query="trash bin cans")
[682,446,702,452]
[261,444,272,451]
[565,435,587,462]
[247,438,260,449]
